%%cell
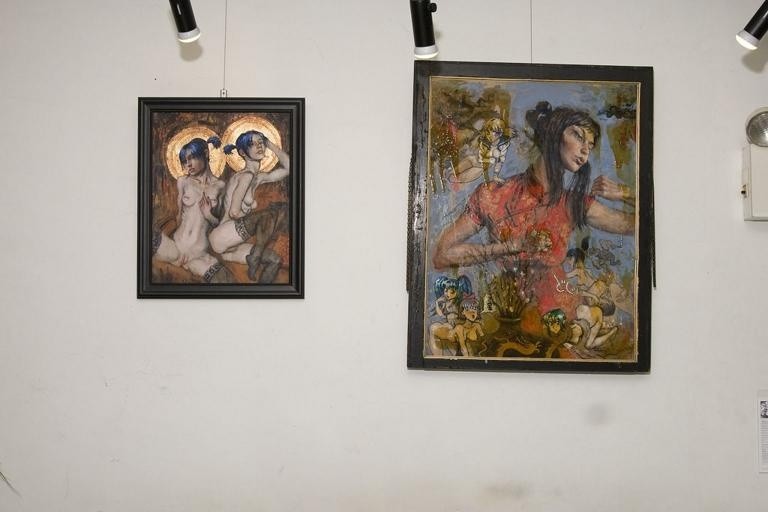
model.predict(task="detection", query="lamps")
[409,0,439,60]
[735,0,768,50]
[168,0,202,43]
[740,105,768,223]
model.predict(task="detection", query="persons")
[208,131,289,285]
[152,137,238,284]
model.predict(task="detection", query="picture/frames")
[407,59,653,374]
[136,97,305,300]
